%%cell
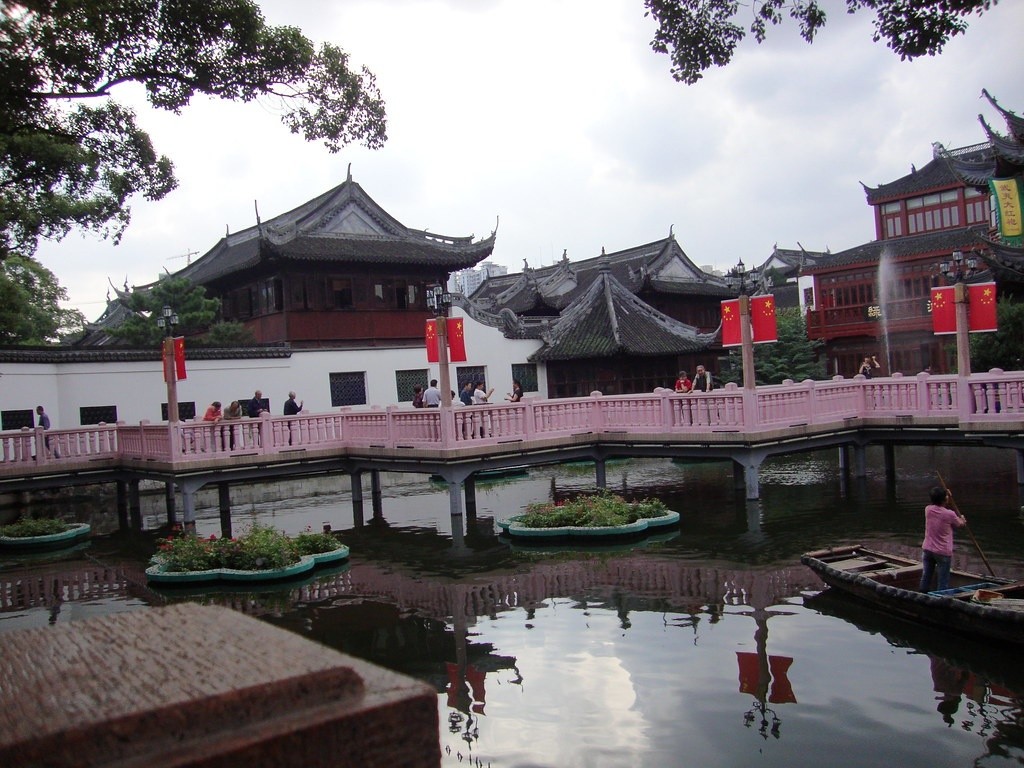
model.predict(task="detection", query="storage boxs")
[928,583,1000,597]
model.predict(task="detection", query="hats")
[679,371,686,376]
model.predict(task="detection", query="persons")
[412,379,494,440]
[687,366,714,394]
[918,487,967,594]
[202,390,268,451]
[505,379,524,402]
[675,371,694,427]
[858,356,880,406]
[29,406,60,459]
[284,392,303,445]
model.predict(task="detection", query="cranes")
[166,249,200,266]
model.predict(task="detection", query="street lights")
[939,247,980,377]
[724,256,759,390]
[426,280,452,407]
[157,304,179,421]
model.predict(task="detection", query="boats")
[800,543,1024,643]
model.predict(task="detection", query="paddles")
[934,469,997,577]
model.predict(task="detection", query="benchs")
[950,583,1023,600]
[861,564,924,592]
